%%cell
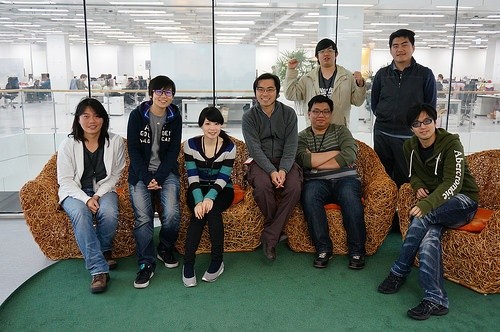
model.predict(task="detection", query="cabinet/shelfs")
[475,94,500,116]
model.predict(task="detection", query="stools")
[3,77,19,108]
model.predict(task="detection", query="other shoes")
[103,251,118,269]
[349,255,365,269]
[201,261,224,282]
[260,236,277,259]
[181,265,197,287]
[314,253,333,267]
[90,273,110,293]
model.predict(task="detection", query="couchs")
[20,137,500,293]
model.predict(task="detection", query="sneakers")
[133,264,154,288]
[378,272,408,294]
[157,248,179,268]
[407,299,449,320]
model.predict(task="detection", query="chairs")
[28,80,40,103]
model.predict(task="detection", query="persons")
[57,98,126,293]
[294,95,366,269]
[76,74,147,105]
[127,76,182,288]
[242,73,303,260]
[371,29,437,234]
[23,74,50,102]
[378,104,479,320]
[438,74,443,83]
[284,38,366,129]
[183,107,237,284]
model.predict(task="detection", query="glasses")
[256,88,277,94]
[311,109,331,115]
[317,50,335,54]
[153,89,173,97]
[411,118,432,128]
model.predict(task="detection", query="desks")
[109,96,124,116]
[438,100,462,127]
[182,99,253,126]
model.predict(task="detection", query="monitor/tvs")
[115,76,126,83]
